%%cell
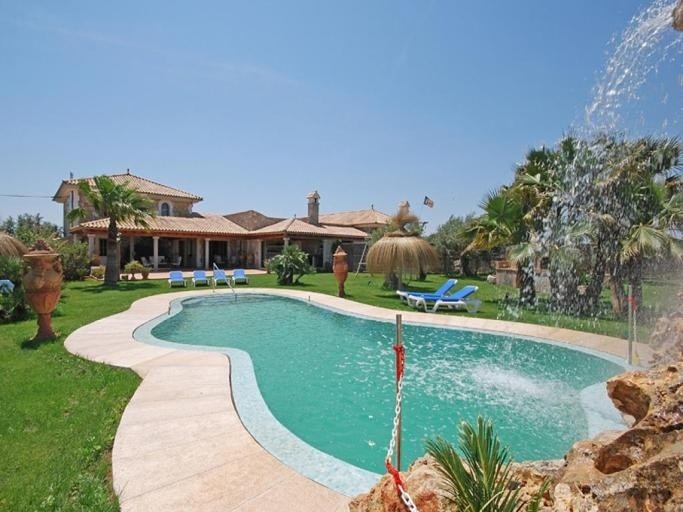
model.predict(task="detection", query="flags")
[423,196,434,209]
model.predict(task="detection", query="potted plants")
[124,260,153,280]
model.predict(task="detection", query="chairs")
[167,269,249,288]
[395,278,482,314]
[140,255,182,266]
